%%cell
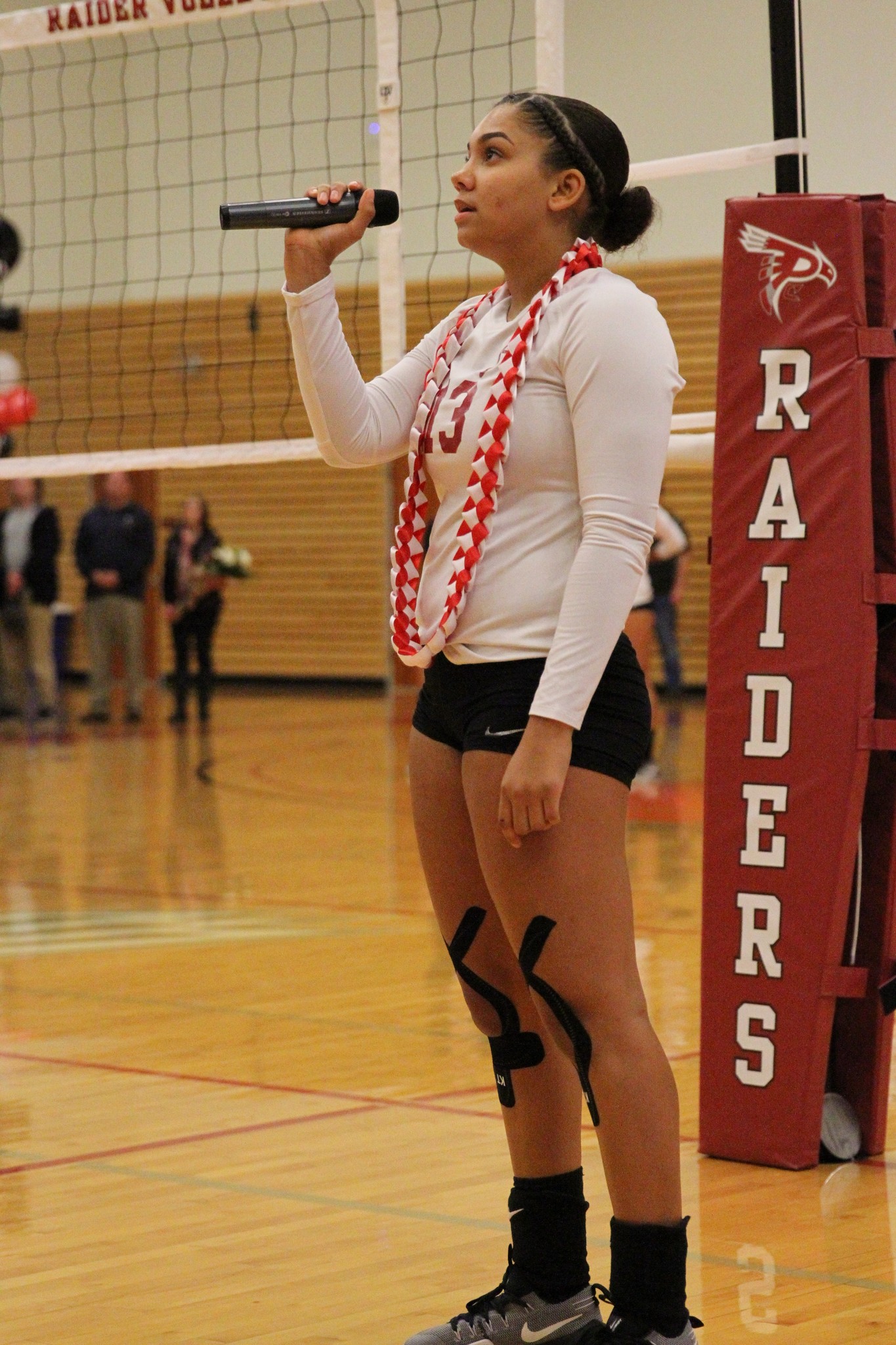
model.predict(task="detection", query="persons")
[0,468,228,742]
[282,91,706,1345]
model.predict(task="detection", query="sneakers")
[404,1243,603,1345]
[537,1283,704,1345]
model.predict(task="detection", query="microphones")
[220,189,399,230]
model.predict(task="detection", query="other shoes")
[198,712,211,722]
[80,712,110,725]
[168,712,188,722]
[122,710,144,723]
